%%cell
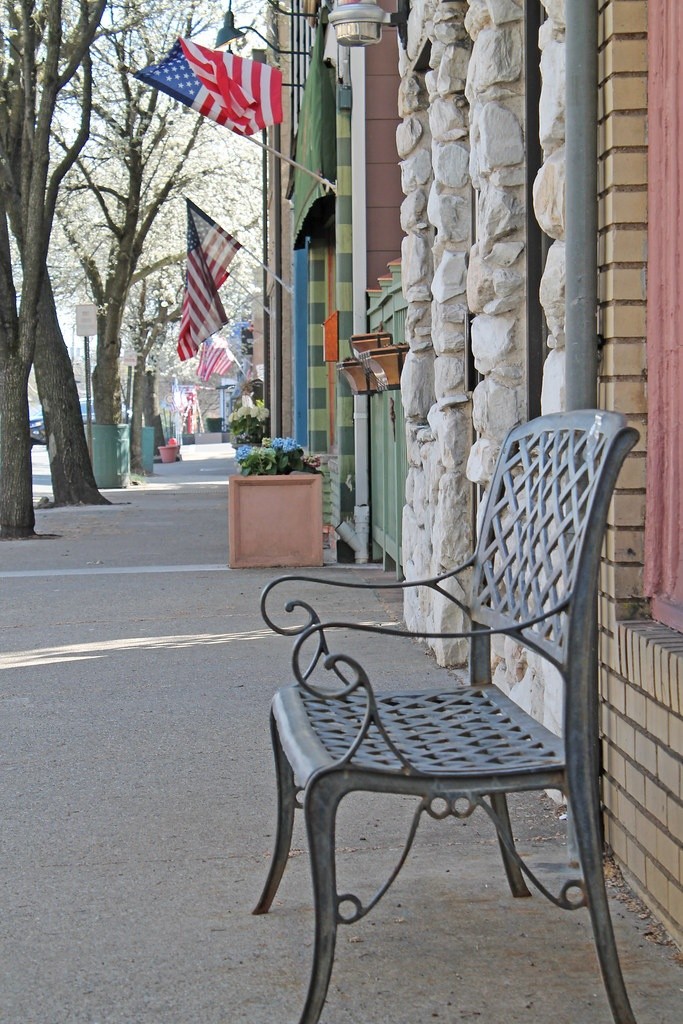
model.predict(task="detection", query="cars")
[30,397,132,449]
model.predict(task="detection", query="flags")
[177,197,242,361]
[196,333,235,382]
[132,37,283,136]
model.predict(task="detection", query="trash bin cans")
[84,423,131,490]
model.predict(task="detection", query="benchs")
[250,408,641,1024]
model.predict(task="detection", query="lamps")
[328,0,410,50]
[214,0,246,48]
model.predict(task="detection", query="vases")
[157,444,178,463]
[348,331,393,360]
[231,441,261,454]
[356,342,410,392]
[335,359,378,396]
[228,470,325,569]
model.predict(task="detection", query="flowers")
[233,436,327,479]
[227,405,270,442]
